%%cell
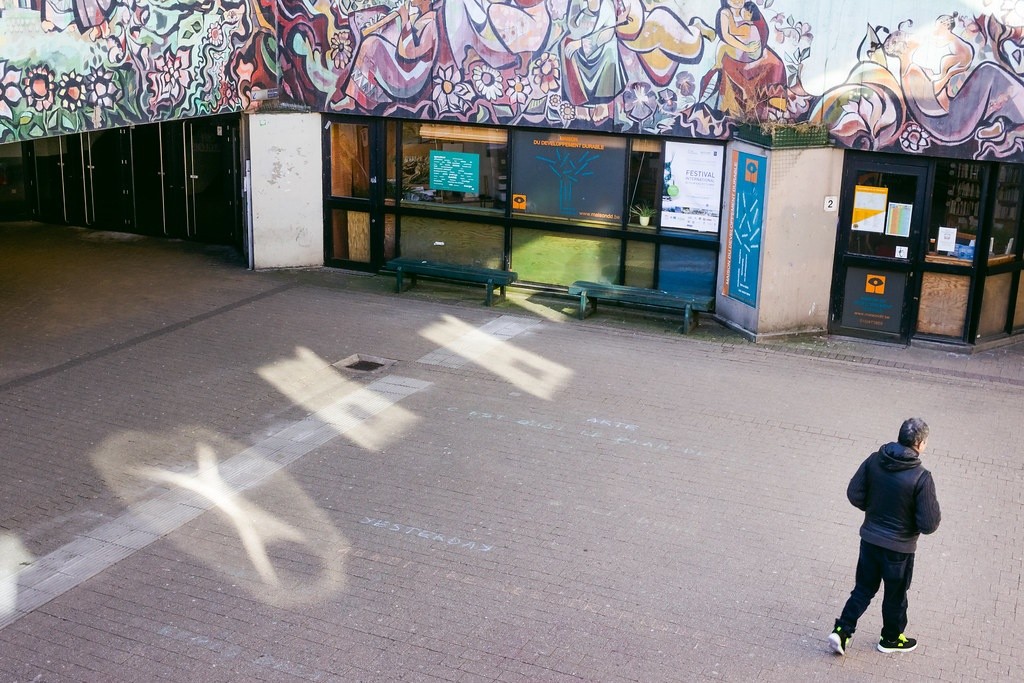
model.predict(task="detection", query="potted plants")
[629,202,657,227]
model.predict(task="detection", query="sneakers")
[828,626,849,655]
[877,635,917,653]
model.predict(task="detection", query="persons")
[827,416,941,656]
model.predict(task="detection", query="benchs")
[388,256,518,308]
[571,280,717,335]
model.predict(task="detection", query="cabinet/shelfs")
[943,159,1024,223]
